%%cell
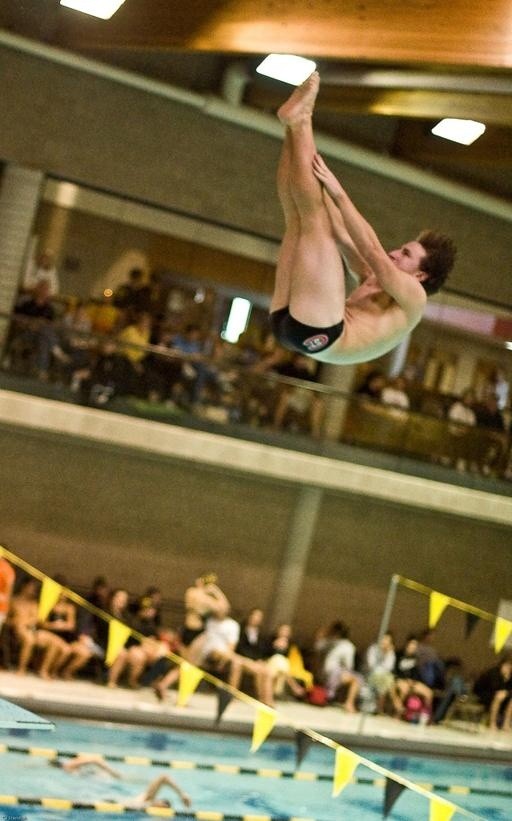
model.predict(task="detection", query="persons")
[10,254,511,479]
[269,70,458,366]
[0,546,512,732]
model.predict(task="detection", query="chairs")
[0,569,511,737]
